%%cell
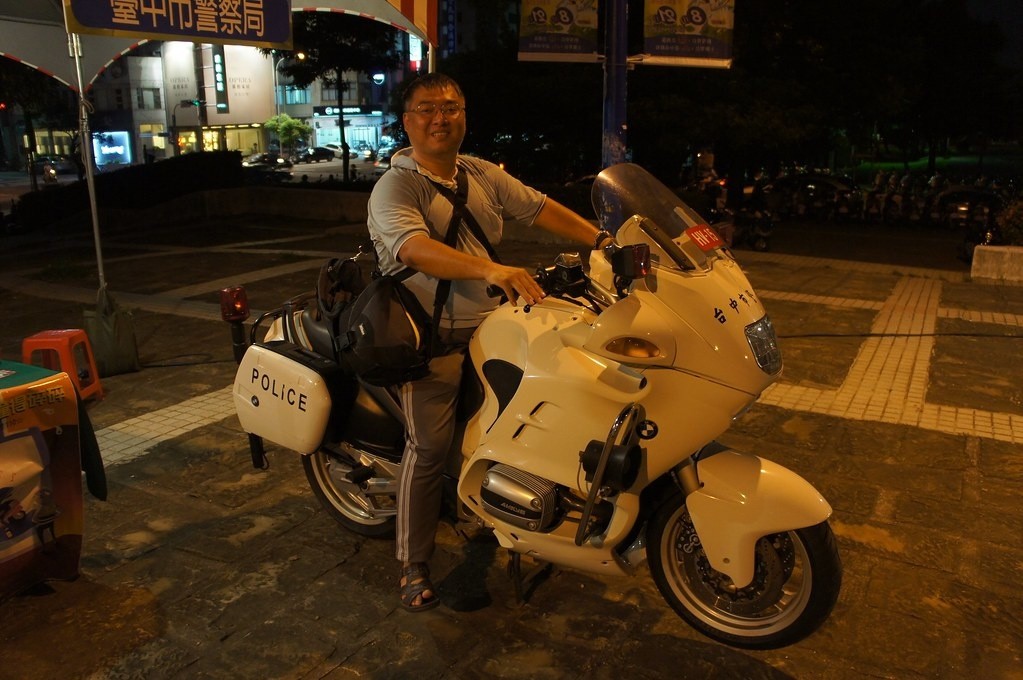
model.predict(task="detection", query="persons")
[142,144,148,163]
[299,174,310,183]
[326,174,336,182]
[43,161,53,175]
[350,164,357,180]
[367,74,611,612]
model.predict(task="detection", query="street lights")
[275,51,305,159]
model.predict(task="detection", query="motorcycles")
[220,164,841,652]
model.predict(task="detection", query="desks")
[0,359,84,604]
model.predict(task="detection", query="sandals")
[396,562,442,612]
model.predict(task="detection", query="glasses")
[405,102,465,121]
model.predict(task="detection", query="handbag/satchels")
[317,258,430,386]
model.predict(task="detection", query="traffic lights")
[191,99,207,106]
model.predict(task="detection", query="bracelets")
[594,230,612,246]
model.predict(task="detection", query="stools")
[21,328,104,403]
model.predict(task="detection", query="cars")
[377,142,404,159]
[326,142,358,160]
[295,146,335,165]
[243,152,293,170]
[354,142,376,161]
[32,155,76,175]
